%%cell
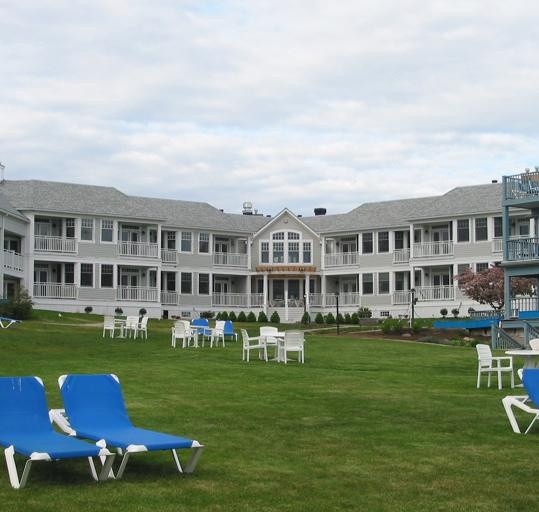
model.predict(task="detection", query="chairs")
[102,315,150,339]
[529,339,539,349]
[476,343,515,389]
[500,369,539,435]
[241,326,306,364]
[0,376,116,490]
[47,374,205,482]
[171,318,238,348]
[0,316,22,329]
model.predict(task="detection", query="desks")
[504,349,539,370]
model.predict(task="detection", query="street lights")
[334,288,340,335]
[304,294,307,313]
[410,288,418,327]
